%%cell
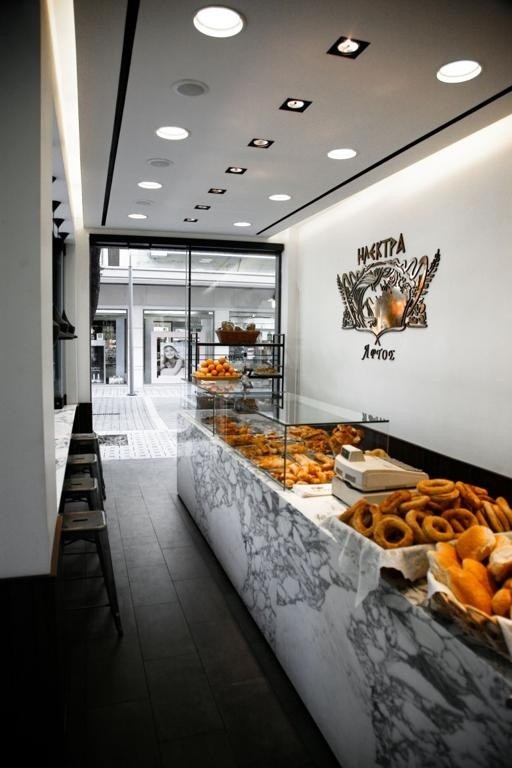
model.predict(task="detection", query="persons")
[160,344,185,376]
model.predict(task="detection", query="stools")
[58,432,124,638]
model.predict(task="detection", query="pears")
[374,285,406,327]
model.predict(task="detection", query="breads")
[341,479,512,619]
[199,383,237,392]
[194,357,240,378]
[256,367,277,374]
[218,320,256,332]
[202,410,388,488]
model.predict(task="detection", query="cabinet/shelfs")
[190,332,285,409]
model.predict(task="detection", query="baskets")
[215,330,260,344]
[429,532,512,662]
